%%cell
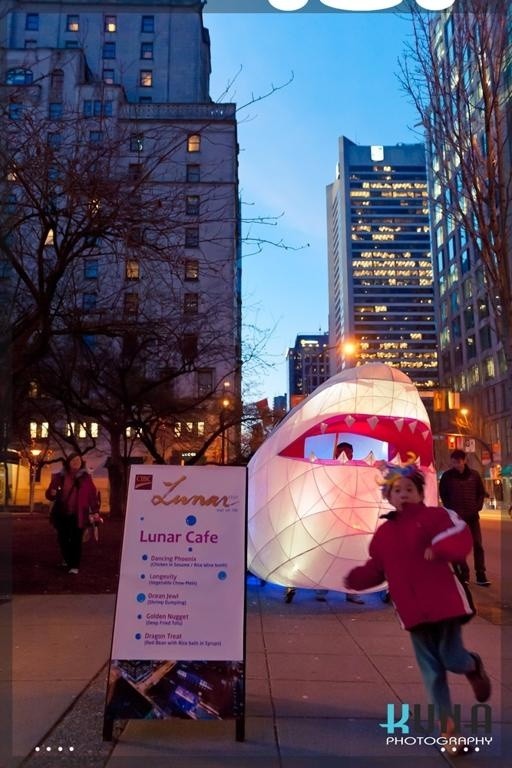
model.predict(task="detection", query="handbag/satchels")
[48,499,70,529]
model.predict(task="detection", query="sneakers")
[476,577,491,585]
[442,733,475,756]
[68,568,78,575]
[465,651,491,702]
[62,560,68,567]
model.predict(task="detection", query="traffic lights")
[447,436,455,449]
[495,464,502,478]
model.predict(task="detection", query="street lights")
[460,407,469,423]
[306,340,357,397]
[29,449,42,512]
[222,398,231,466]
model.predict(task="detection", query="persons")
[344,453,491,757]
[438,448,493,586]
[45,452,101,577]
